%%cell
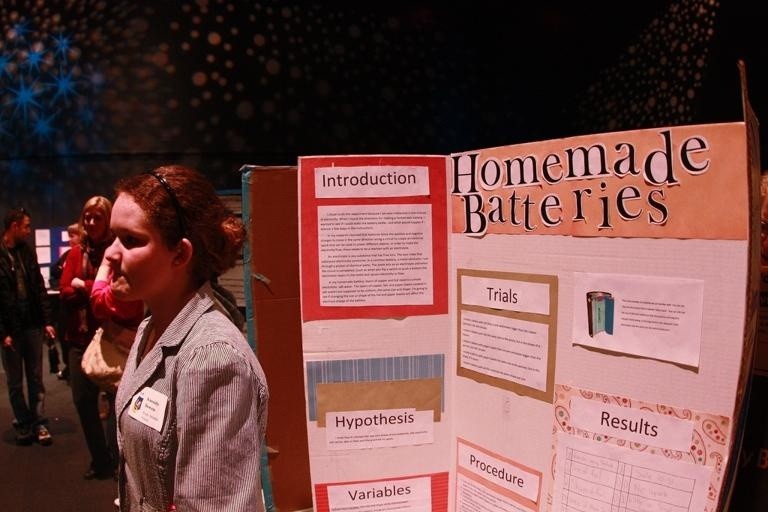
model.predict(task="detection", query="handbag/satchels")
[81,327,131,394]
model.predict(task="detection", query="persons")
[44,248,71,375]
[57,219,87,380]
[102,162,272,512]
[58,194,118,479]
[0,205,57,446]
[88,238,152,509]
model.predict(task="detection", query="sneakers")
[35,425,51,441]
[16,428,32,439]
